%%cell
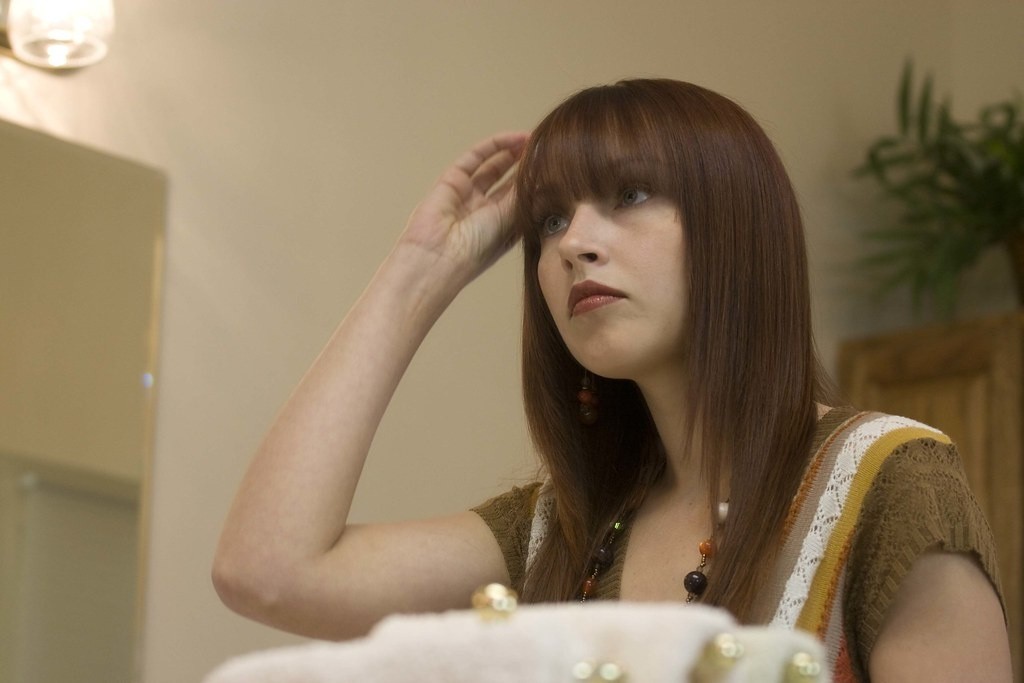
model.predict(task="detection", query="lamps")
[0,0,116,68]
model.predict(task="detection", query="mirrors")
[0,120,170,683]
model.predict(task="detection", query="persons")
[210,79,1015,683]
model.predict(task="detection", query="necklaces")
[572,483,731,604]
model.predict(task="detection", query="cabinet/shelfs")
[838,308,1024,683]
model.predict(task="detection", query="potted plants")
[849,54,1024,332]
